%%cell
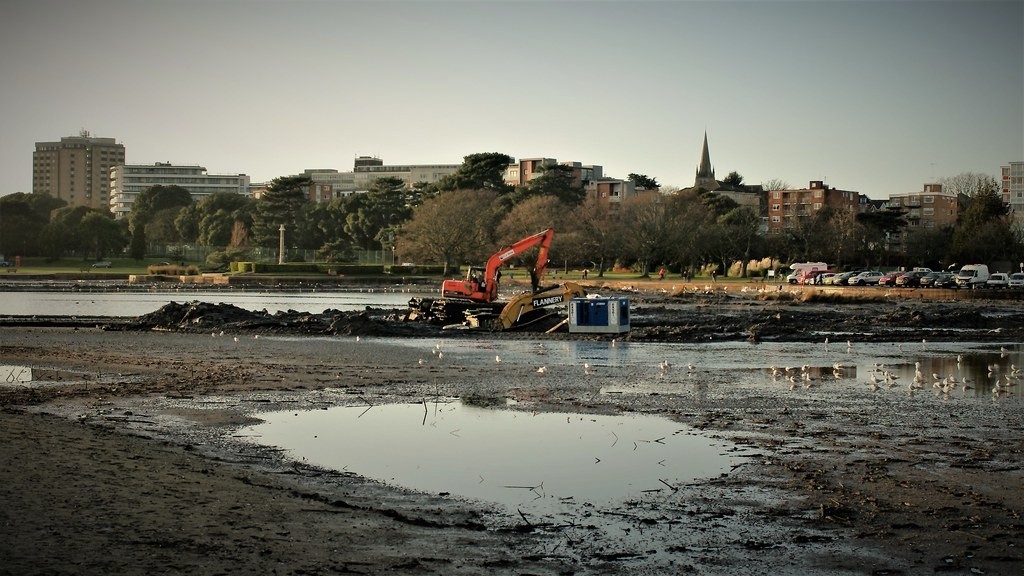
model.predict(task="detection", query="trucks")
[786,262,829,283]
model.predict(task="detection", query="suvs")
[987,272,1024,290]
[801,268,955,289]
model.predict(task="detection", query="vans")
[956,263,988,289]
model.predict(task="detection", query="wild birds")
[768,361,1024,402]
[356,335,360,341]
[495,355,502,363]
[987,327,1006,334]
[536,366,546,372]
[847,340,855,348]
[621,285,729,295]
[1000,346,1009,352]
[825,338,829,344]
[659,359,677,371]
[584,360,590,375]
[1000,352,1009,358]
[687,362,697,370]
[210,330,263,343]
[611,339,616,348]
[740,286,803,295]
[922,338,928,351]
[957,353,964,366]
[418,342,444,365]
[899,344,904,354]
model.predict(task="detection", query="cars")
[92,260,112,267]
[150,261,170,265]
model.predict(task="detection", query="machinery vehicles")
[410,228,588,334]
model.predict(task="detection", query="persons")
[711,271,717,283]
[683,269,691,283]
[813,273,823,286]
[779,269,784,279]
[509,271,514,279]
[471,270,485,287]
[658,268,665,281]
